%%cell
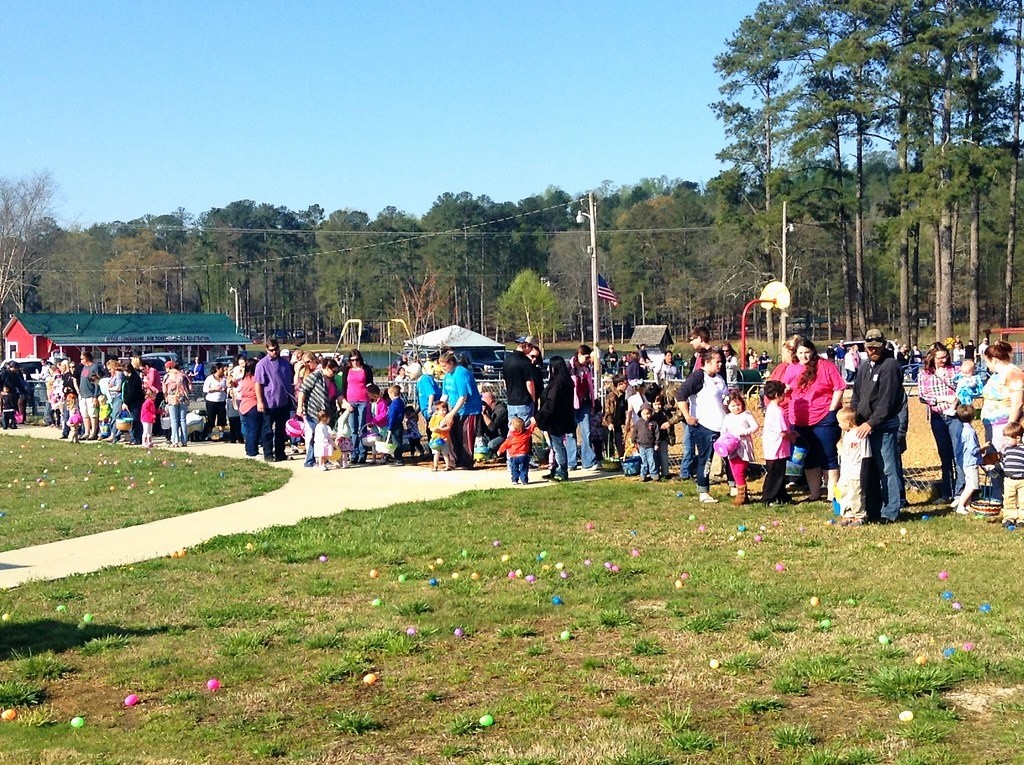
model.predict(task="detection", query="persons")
[0,326,1024,529]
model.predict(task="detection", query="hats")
[865,329,885,349]
[10,362,18,366]
[515,336,534,344]
[165,361,173,367]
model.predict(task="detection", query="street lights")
[228,286,240,334]
[576,190,599,352]
[780,200,795,360]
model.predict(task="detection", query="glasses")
[529,354,539,358]
[584,356,591,359]
[305,359,312,364]
[689,337,697,343]
[69,366,76,369]
[81,358,84,361]
[268,347,278,351]
[330,368,338,373]
[194,358,199,361]
[351,356,362,362]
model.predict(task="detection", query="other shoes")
[838,518,865,527]
[1000,519,1024,530]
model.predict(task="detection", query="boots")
[727,485,754,506]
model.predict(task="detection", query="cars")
[266,327,287,339]
[292,330,305,338]
[236,326,259,341]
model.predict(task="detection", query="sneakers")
[542,471,569,484]
[59,431,202,448]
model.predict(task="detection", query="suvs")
[388,348,549,381]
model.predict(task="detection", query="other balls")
[584,490,1017,721]
[0,543,576,729]
[0,438,226,515]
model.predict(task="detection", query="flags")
[596,273,618,307]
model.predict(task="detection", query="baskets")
[359,423,399,458]
[600,429,642,476]
[969,473,1004,518]
[114,407,134,431]
